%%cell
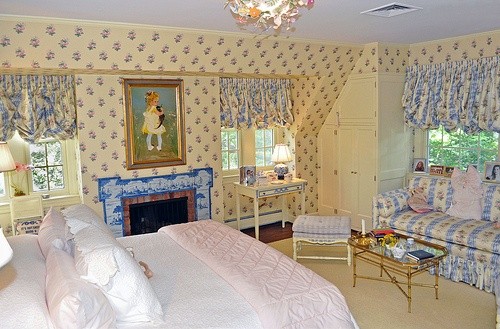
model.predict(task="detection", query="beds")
[0,219,360,329]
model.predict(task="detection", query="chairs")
[10,195,45,236]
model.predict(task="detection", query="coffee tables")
[348,233,448,314]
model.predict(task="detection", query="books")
[407,250,435,262]
[371,227,396,240]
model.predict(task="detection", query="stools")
[291,214,353,267]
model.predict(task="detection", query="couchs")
[371,177,500,295]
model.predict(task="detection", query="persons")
[486,165,500,181]
[414,161,425,172]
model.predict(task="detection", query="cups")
[285,173,292,182]
[407,238,414,244]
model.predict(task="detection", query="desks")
[232,177,307,240]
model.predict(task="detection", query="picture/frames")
[483,161,500,183]
[121,77,187,171]
[444,166,454,178]
[413,158,426,173]
[429,165,445,176]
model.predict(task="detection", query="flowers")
[9,162,35,194]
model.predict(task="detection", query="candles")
[361,218,366,234]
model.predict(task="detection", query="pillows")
[45,238,119,329]
[72,225,167,328]
[446,164,484,220]
[60,202,116,240]
[36,205,75,259]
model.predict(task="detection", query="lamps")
[0,141,17,173]
[270,143,293,180]
[224,0,315,39]
